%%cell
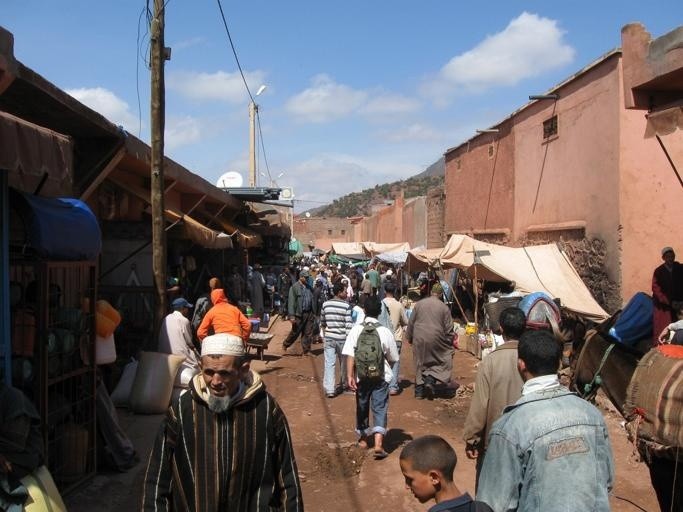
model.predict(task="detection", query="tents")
[304,237,444,265]
[442,233,613,325]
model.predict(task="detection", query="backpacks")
[356,320,386,383]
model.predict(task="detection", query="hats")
[172,298,194,309]
[662,247,674,255]
[432,282,442,293]
[200,333,244,357]
[299,270,311,278]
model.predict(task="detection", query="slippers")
[373,450,388,457]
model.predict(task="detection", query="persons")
[158,298,198,368]
[250,264,265,315]
[475,329,614,512]
[378,276,399,299]
[282,270,318,358]
[651,246,683,319]
[399,435,494,512]
[190,278,219,330]
[463,307,527,498]
[0,383,45,512]
[277,267,292,318]
[312,280,328,344]
[361,274,373,295]
[341,296,399,458]
[657,319,683,345]
[139,332,304,512]
[290,253,362,290]
[227,264,245,303]
[320,283,354,398]
[405,283,455,401]
[196,288,252,346]
[379,283,409,396]
[367,267,380,292]
[408,277,428,299]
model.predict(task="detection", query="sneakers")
[390,387,398,394]
[415,392,423,400]
[425,384,434,400]
